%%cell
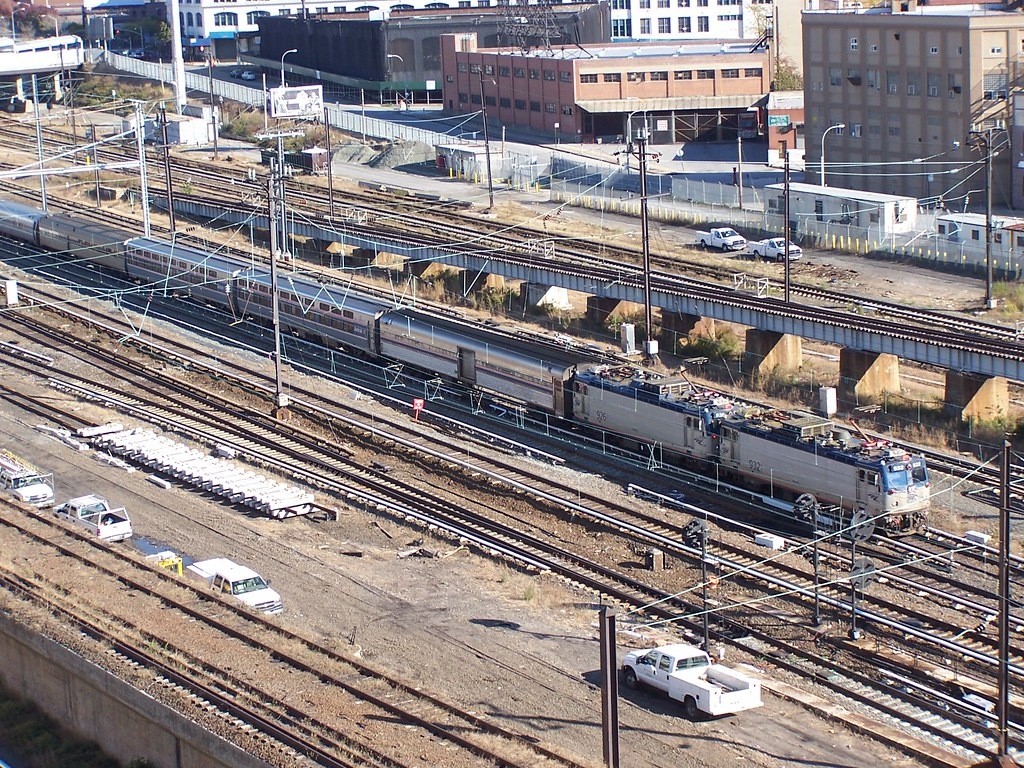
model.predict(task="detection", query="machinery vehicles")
[0,448,55,508]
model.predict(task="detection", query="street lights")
[627,110,648,174]
[387,54,408,113]
[821,124,845,187]
[282,48,297,88]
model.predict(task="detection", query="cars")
[241,71,256,81]
[229,68,241,78]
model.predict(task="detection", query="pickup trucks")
[51,494,133,543]
[621,643,765,722]
[185,558,284,618]
[695,227,746,252]
[746,236,803,261]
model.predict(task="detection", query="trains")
[1,199,930,537]
